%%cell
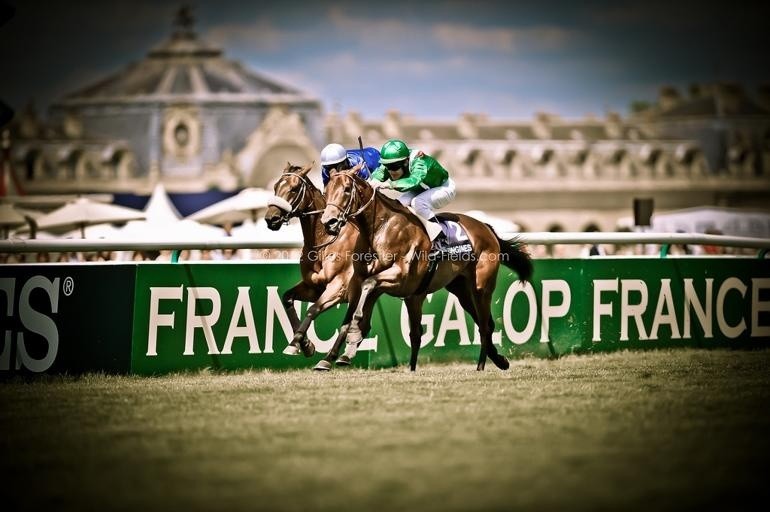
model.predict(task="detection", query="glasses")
[383,164,404,171]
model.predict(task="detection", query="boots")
[428,216,449,260]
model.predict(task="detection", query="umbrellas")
[13,195,147,242]
[181,180,278,240]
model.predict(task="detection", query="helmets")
[319,143,350,166]
[378,139,411,164]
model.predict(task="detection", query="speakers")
[632,196,653,227]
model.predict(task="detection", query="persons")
[318,142,381,190]
[366,138,458,262]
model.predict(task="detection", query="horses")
[264,160,538,373]
